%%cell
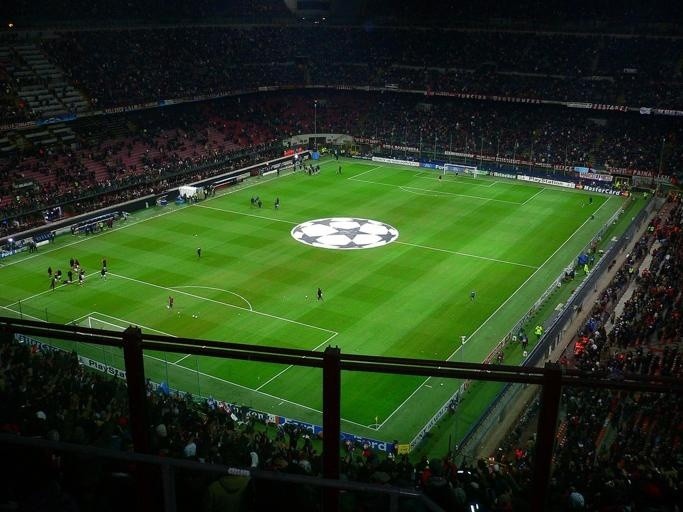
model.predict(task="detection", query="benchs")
[1,329,589,512]
[508,188,682,512]
[313,27,683,189]
[2,27,313,236]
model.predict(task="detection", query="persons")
[316,288,323,302]
[0,322,424,508]
[1,1,317,292]
[316,1,682,185]
[168,296,173,311]
[425,185,682,510]
